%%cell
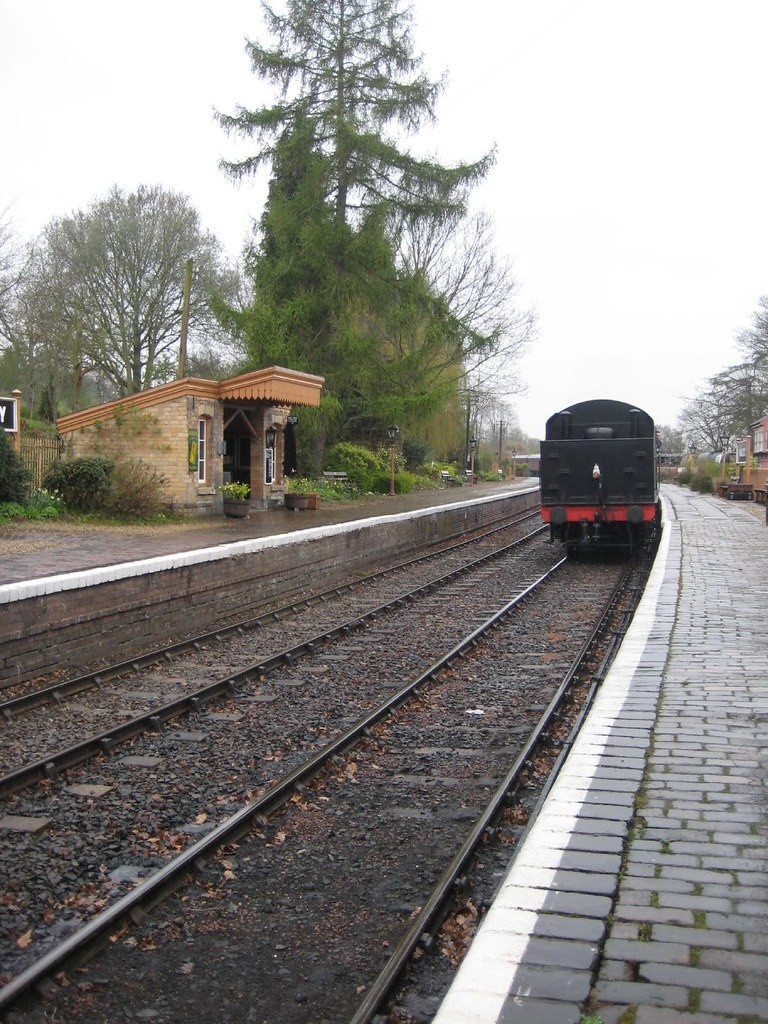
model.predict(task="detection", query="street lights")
[469,436,478,486]
[689,444,697,474]
[386,423,401,496]
[512,448,518,478]
[719,429,729,481]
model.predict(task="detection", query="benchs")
[323,472,358,486]
[466,470,478,484]
[441,471,457,484]
[754,481,768,504]
[720,476,741,498]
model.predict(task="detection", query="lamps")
[266,426,279,448]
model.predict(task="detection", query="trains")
[537,398,661,559]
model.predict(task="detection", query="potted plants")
[217,481,254,518]
[285,478,309,513]
